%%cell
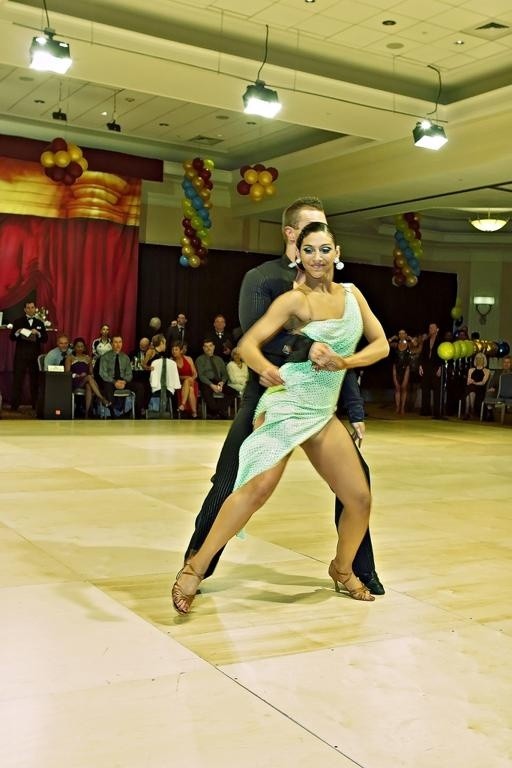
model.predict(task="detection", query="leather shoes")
[356,570,385,596]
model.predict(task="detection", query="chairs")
[37,352,254,419]
[458,368,512,425]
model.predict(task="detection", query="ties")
[209,355,220,378]
[179,327,184,342]
[113,353,121,381]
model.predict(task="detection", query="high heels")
[171,559,204,615]
[329,560,374,602]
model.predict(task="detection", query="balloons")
[237,164,279,201]
[392,213,424,288]
[437,339,510,361]
[179,157,215,269]
[40,139,88,186]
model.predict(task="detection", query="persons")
[181,195,385,596]
[41,313,491,423]
[171,221,390,616]
[9,300,48,412]
[492,356,512,389]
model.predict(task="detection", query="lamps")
[469,212,511,234]
[412,63,449,151]
[27,0,73,75]
[242,24,281,120]
